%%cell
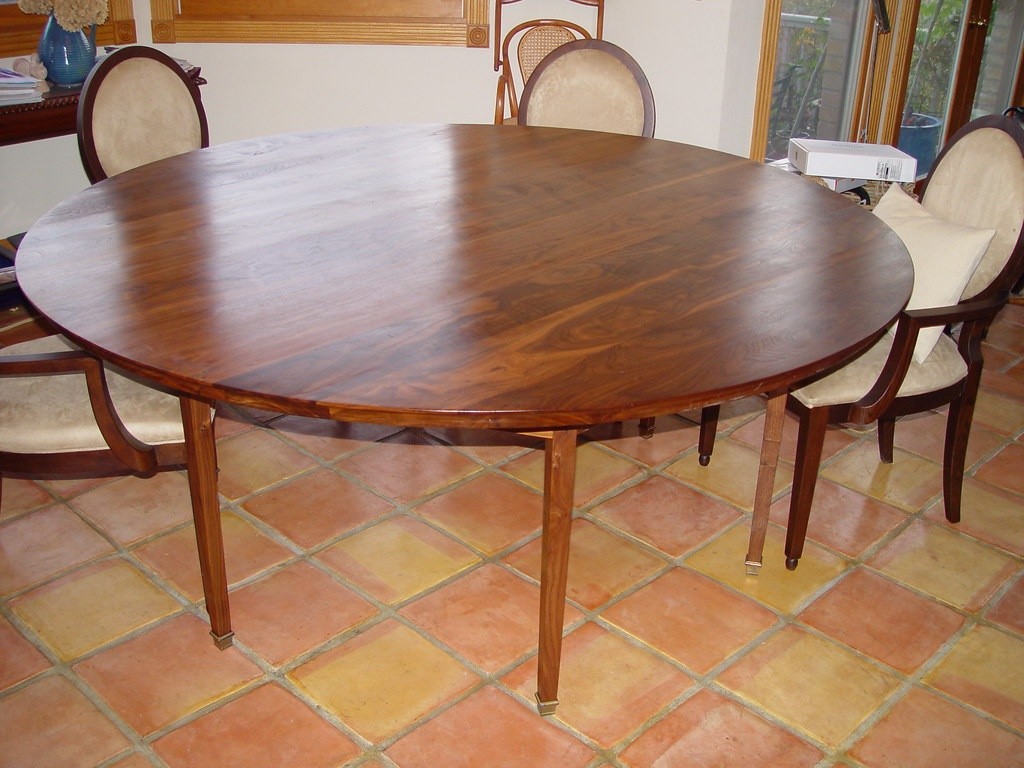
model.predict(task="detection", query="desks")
[0,64,202,147]
[21,123,916,718]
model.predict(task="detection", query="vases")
[38,9,94,88]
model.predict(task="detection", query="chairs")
[76,44,209,200]
[0,259,219,533]
[698,111,1024,569]
[492,0,605,122]
[517,36,655,141]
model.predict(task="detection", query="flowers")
[15,0,110,32]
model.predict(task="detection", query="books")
[0,232,28,272]
[0,68,48,107]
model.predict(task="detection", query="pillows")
[866,180,995,364]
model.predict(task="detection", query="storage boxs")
[763,157,869,193]
[787,137,918,184]
